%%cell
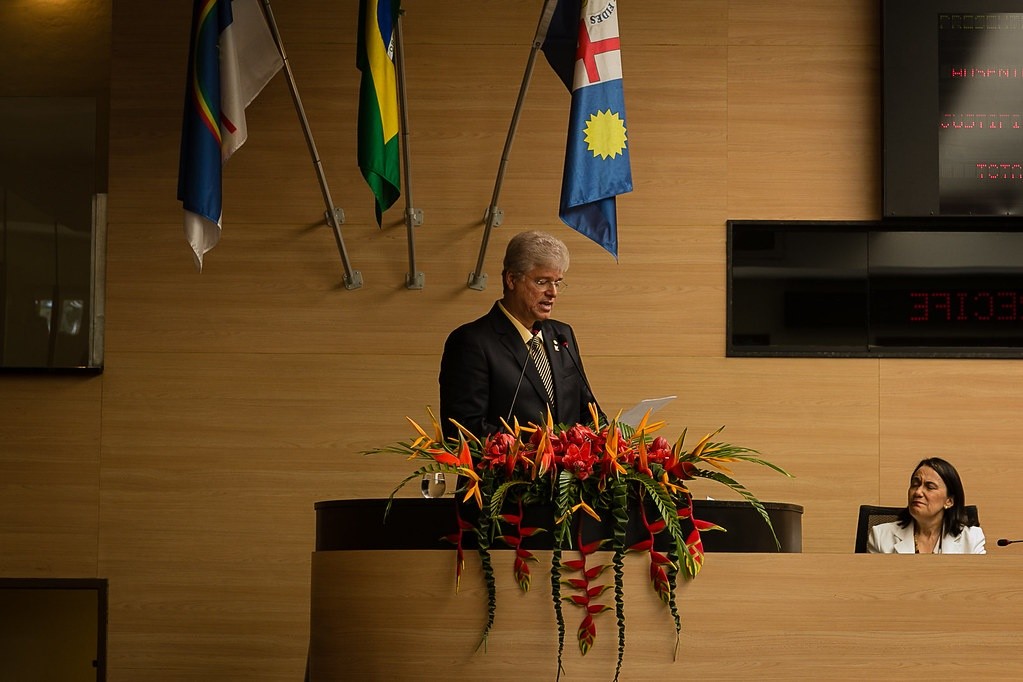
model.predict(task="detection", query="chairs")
[855,505,980,554]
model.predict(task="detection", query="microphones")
[502,320,544,433]
[997,539,1023,546]
[557,333,611,427]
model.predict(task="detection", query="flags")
[176,0,287,275]
[353,1,403,229]
[530,0,632,263]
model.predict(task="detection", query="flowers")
[356,402,797,682]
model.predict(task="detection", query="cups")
[421,473,445,498]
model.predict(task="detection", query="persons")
[438,232,609,500]
[866,457,988,555]
